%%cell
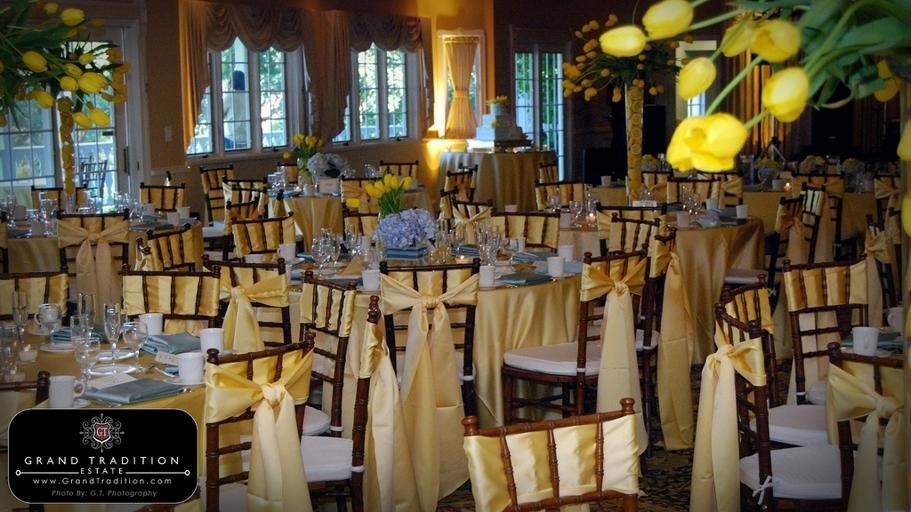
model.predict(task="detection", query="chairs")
[0,147,911,512]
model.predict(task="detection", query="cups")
[557,244,574,266]
[175,352,209,384]
[772,179,785,192]
[240,244,296,265]
[199,328,228,358]
[138,312,163,336]
[887,308,904,332]
[47,375,84,409]
[546,256,566,276]
[851,325,880,357]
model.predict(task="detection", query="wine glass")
[543,176,749,231]
[0,290,153,385]
[0,187,191,237]
[310,216,528,294]
[265,153,383,198]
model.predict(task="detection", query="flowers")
[560,0,911,239]
[282,133,324,160]
[0,0,130,197]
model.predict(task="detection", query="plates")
[842,347,892,359]
[544,272,575,277]
[878,327,899,334]
[162,374,207,389]
[289,257,305,266]
[39,398,91,409]
[187,348,238,356]
